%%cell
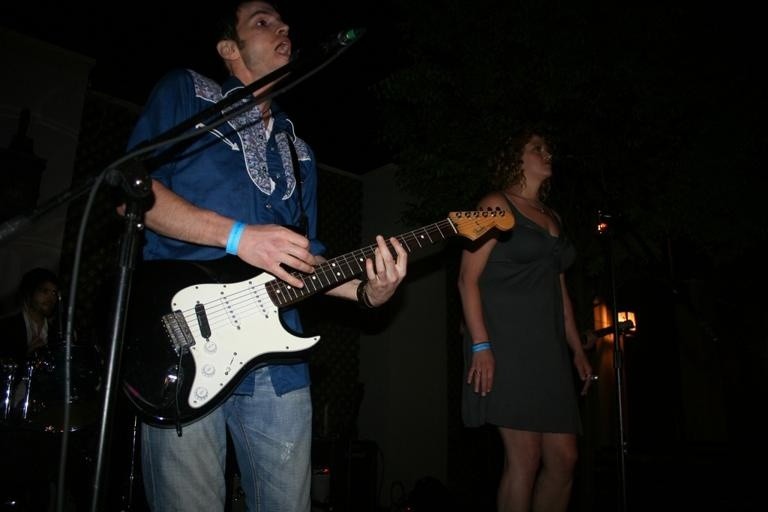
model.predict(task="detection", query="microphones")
[288,27,358,68]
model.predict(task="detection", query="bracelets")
[470,341,492,353]
[357,279,375,312]
[225,220,244,256]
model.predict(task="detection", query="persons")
[117,1,409,512]
[0,266,63,365]
[454,126,593,512]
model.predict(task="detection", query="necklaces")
[511,193,545,212]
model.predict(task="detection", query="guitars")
[94,206,515,429]
